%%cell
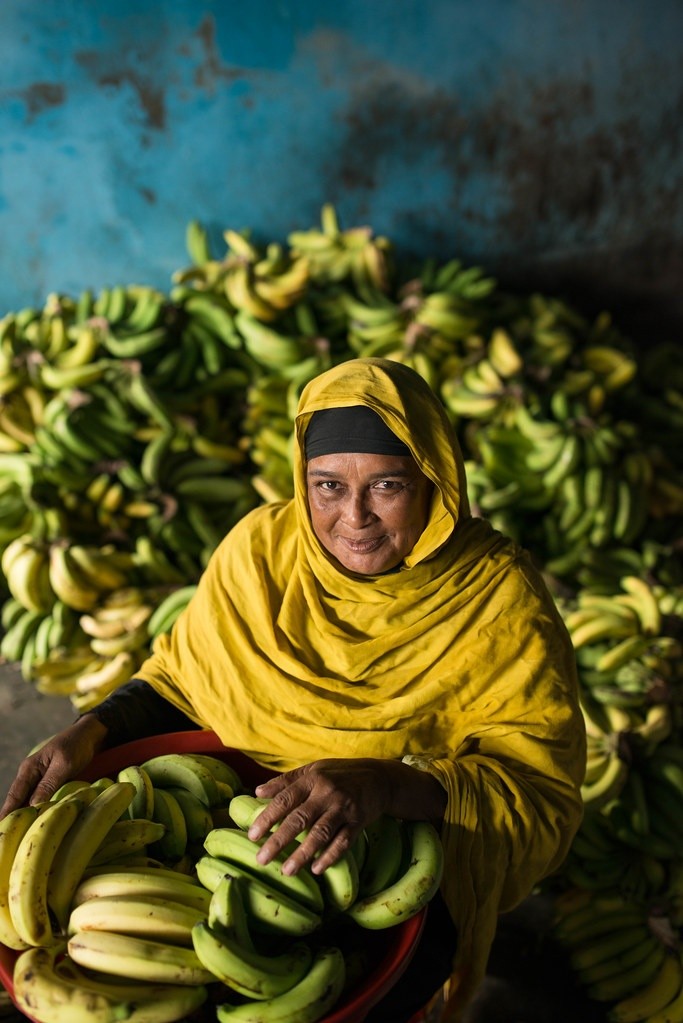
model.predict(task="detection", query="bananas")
[0,201,680,1022]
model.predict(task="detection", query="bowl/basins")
[2,730,426,1023]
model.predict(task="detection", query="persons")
[0,350,590,920]
